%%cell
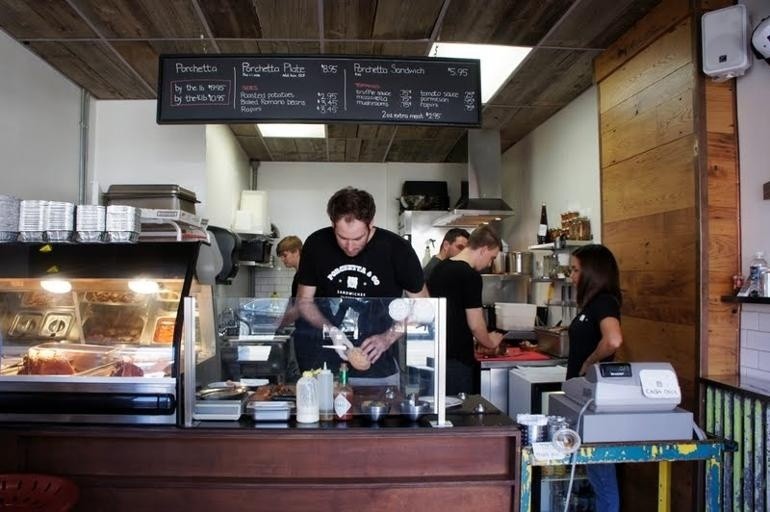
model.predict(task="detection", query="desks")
[507,435,726,512]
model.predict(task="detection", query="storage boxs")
[105,184,201,233]
[495,302,537,331]
[237,233,273,263]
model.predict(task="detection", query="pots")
[491,253,506,274]
[508,251,531,275]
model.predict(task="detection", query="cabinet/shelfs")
[0,229,225,426]
[526,240,593,308]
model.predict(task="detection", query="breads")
[347,348,371,371]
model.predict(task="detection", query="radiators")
[705,381,770,511]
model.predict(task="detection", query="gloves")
[328,325,354,362]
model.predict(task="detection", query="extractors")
[431,129,515,227]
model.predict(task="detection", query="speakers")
[701,4,752,82]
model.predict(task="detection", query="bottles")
[750,252,769,297]
[542,253,559,278]
[297,362,353,423]
[423,246,431,266]
[537,204,550,244]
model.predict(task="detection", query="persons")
[564,244,624,511]
[297,186,430,385]
[276,236,321,375]
[425,229,503,395]
[423,228,471,298]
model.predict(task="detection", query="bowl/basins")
[400,401,430,420]
[360,400,391,421]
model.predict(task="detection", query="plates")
[418,395,461,408]
[198,388,247,399]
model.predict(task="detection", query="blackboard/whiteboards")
[157,53,482,127]
